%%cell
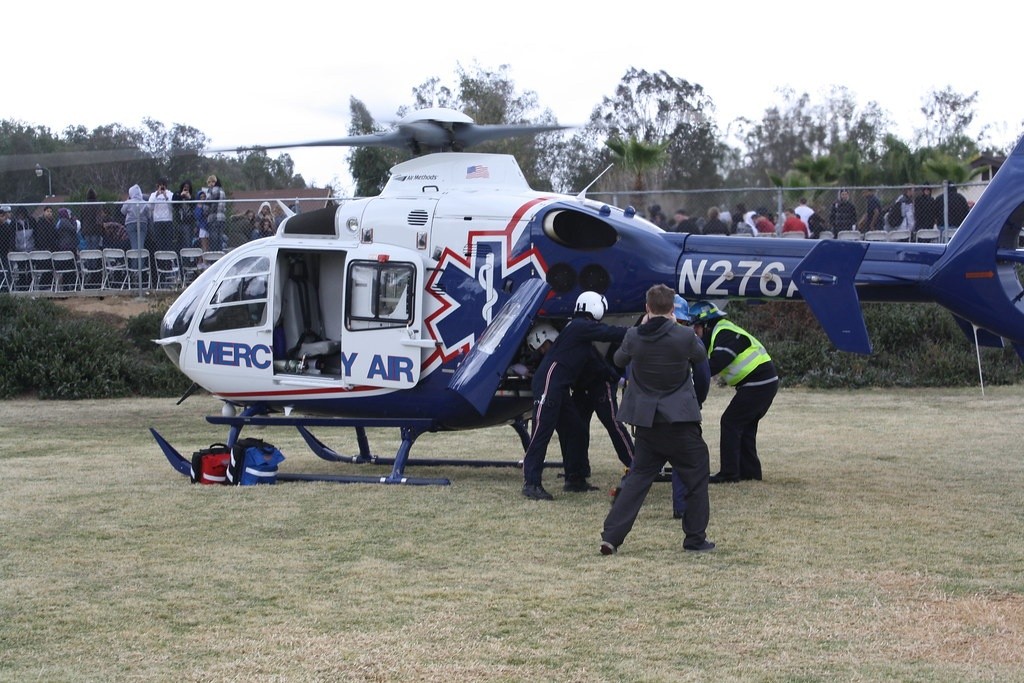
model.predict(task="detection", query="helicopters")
[0,119,1024,485]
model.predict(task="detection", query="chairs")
[0,247,226,292]
[730,228,957,244]
[282,262,340,359]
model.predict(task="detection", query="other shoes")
[684,541,715,550]
[601,541,618,555]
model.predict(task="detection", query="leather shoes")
[709,472,740,483]
[522,484,553,500]
[564,484,599,492]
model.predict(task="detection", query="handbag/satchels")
[191,437,285,485]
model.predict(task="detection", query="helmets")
[526,323,559,353]
[686,301,727,326]
[574,291,608,321]
[673,294,691,320]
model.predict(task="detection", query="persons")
[0,176,296,293]
[647,181,975,243]
[521,285,779,556]
